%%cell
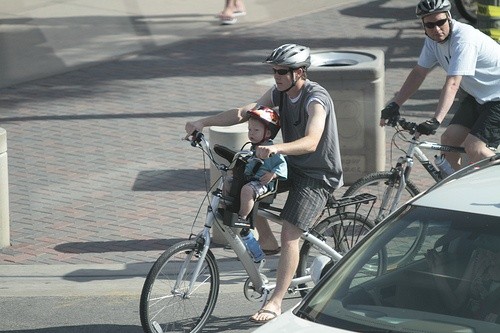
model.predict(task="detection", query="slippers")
[236,245,282,261]
[249,308,278,323]
[217,9,246,25]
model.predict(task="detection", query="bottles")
[239,227,266,262]
[433,154,456,178]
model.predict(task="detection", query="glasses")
[273,68,294,75]
[423,15,449,28]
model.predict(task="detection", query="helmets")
[248,105,281,128]
[266,44,310,69]
[416,0,451,19]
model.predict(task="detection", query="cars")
[250,151,500,333]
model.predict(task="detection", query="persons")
[425,249,500,324]
[220,0,246,24]
[183,43,343,324]
[218,105,288,223]
[380,0,500,170]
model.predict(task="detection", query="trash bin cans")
[306,48,386,185]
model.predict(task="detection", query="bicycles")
[333,111,500,273]
[139,128,388,333]
[454,0,479,23]
